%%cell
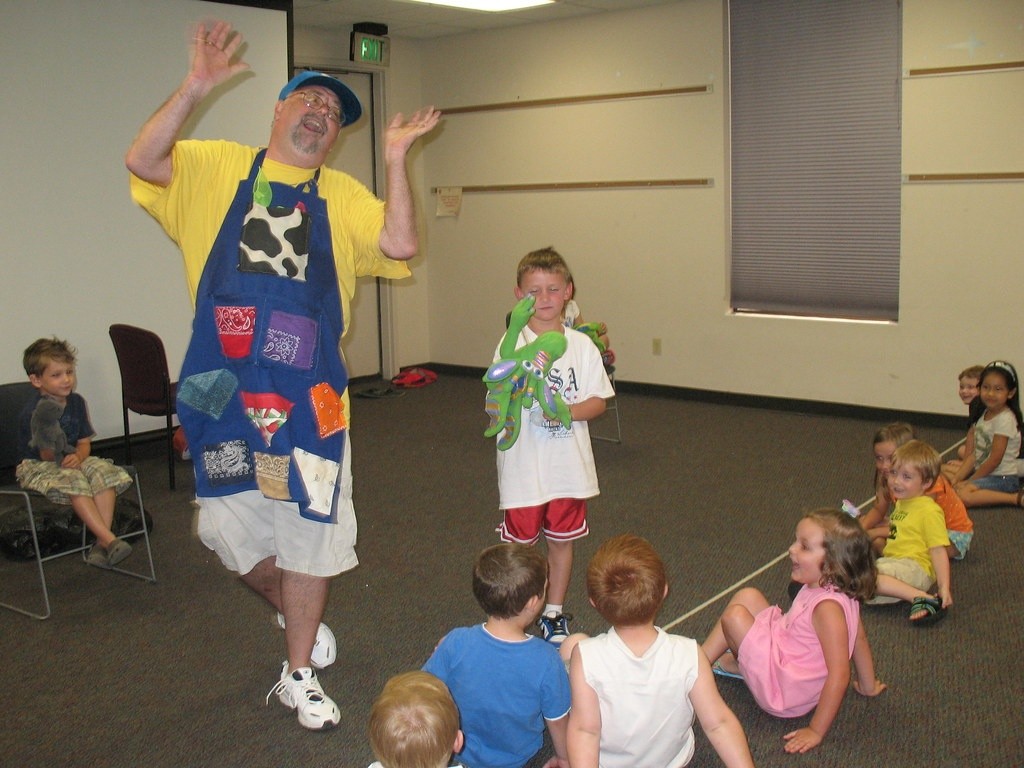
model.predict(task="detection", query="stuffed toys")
[573,323,607,353]
[481,292,572,451]
[27,398,77,466]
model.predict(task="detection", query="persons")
[12,338,133,569]
[366,671,465,767]
[699,510,887,754]
[484,247,616,647]
[122,20,442,731]
[942,359,1024,506]
[858,422,974,560]
[558,534,755,768]
[421,542,571,768]
[788,440,953,624]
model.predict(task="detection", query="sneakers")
[536,609,573,649]
[264,660,341,731]
[277,609,337,670]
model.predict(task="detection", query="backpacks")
[392,367,438,388]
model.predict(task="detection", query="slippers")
[88,537,133,570]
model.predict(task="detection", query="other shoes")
[1016,488,1023,508]
[356,380,406,399]
[712,650,745,682]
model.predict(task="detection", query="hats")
[279,71,362,127]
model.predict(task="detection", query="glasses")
[283,92,347,125]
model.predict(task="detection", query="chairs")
[108,323,178,491]
[505,311,623,445]
[0,382,157,620]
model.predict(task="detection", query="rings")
[205,40,214,45]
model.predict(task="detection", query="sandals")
[908,595,949,625]
